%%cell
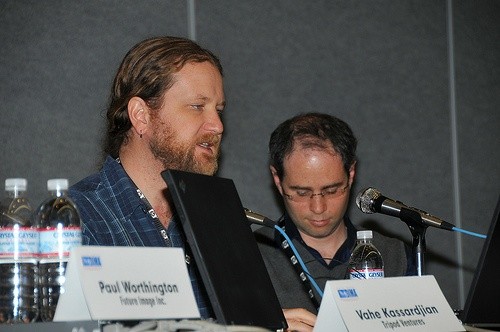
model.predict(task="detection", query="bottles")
[0,178,40,323]
[349,231,385,279]
[35,179,82,322]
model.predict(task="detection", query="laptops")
[452,198,499,332]
[159,168,289,331]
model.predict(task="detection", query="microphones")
[244,207,277,228]
[355,187,456,232]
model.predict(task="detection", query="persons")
[62,34,226,320]
[255,112,407,332]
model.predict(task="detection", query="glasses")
[280,177,349,202]
